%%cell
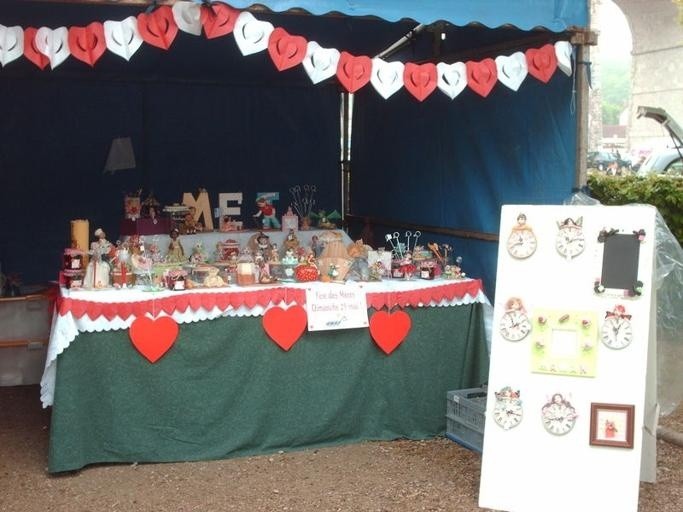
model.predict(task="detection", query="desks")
[41,224,494,476]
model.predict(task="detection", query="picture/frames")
[588,401,634,449]
[527,307,599,379]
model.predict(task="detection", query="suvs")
[632,104,683,177]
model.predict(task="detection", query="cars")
[587,151,631,171]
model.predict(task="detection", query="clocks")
[541,390,579,435]
[553,215,586,257]
[493,385,522,429]
[600,305,633,349]
[506,214,537,259]
[498,298,531,341]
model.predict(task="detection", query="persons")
[220,215,241,232]
[413,245,424,257]
[252,197,282,230]
[184,206,202,234]
[271,228,387,281]
[400,250,416,281]
[83,228,273,292]
[434,263,461,280]
